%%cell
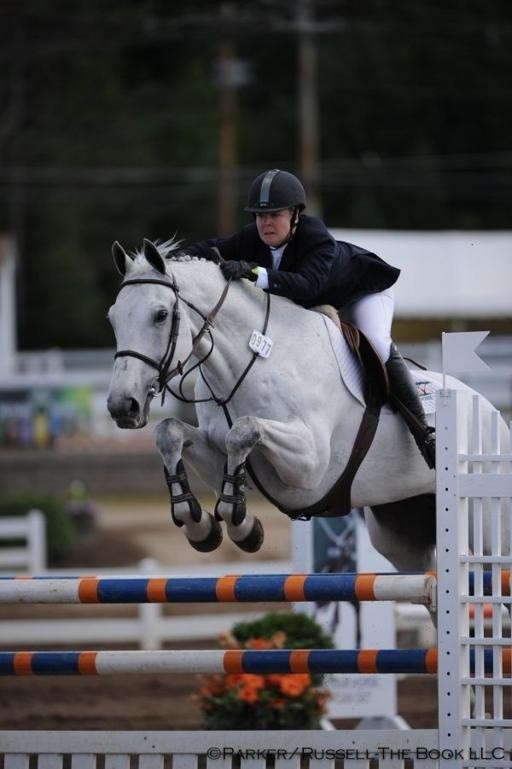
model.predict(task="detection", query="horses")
[106,230,512,640]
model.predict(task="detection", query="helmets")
[243,167,307,213]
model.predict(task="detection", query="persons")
[166,165,438,471]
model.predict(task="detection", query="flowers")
[195,608,329,732]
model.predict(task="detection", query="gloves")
[219,259,259,282]
[178,241,212,264]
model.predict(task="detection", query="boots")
[381,339,436,470]
